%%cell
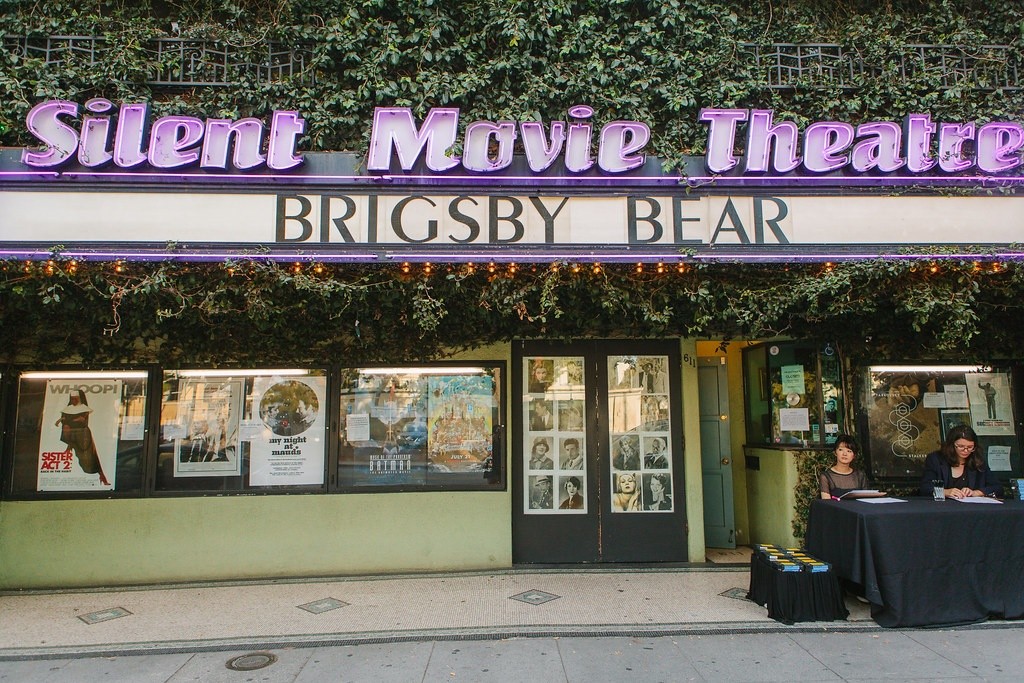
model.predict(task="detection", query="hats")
[641,361,654,369]
[535,476,551,486]
[532,436,549,453]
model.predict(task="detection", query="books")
[838,490,886,500]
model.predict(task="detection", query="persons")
[819,434,866,499]
[186,412,224,463]
[266,383,318,435]
[922,424,1004,499]
[529,357,673,510]
[55,390,111,486]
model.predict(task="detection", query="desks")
[804,499,1024,629]
[747,549,851,626]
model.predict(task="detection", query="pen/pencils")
[831,495,841,501]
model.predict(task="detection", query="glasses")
[954,444,975,452]
